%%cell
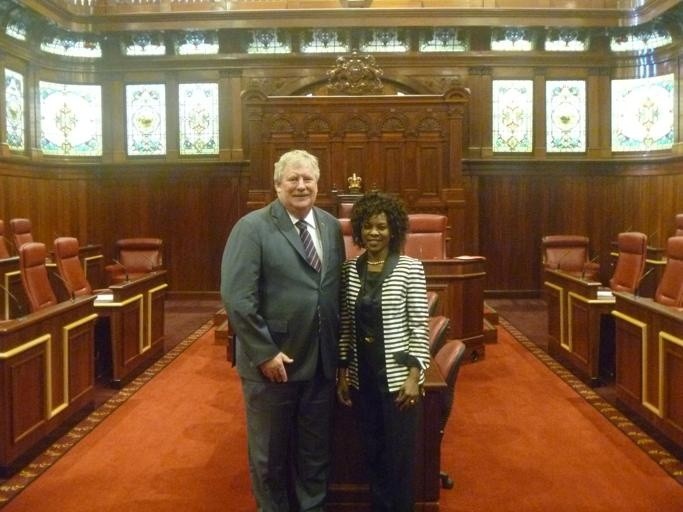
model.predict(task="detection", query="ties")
[295,221,321,272]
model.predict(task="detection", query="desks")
[227,320,447,512]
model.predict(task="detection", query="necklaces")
[366,258,384,266]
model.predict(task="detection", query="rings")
[410,399,415,405]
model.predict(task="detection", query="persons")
[335,189,431,511]
[219,150,344,512]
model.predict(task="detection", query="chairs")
[540,212,683,308]
[0,216,163,313]
[426,290,467,490]
[336,202,452,261]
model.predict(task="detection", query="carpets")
[0,304,683,512]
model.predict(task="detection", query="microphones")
[52,272,76,303]
[112,258,130,282]
[3,237,21,257]
[625,225,634,232]
[634,267,655,300]
[0,284,27,322]
[581,255,600,279]
[53,231,60,238]
[143,255,156,275]
[647,230,658,239]
